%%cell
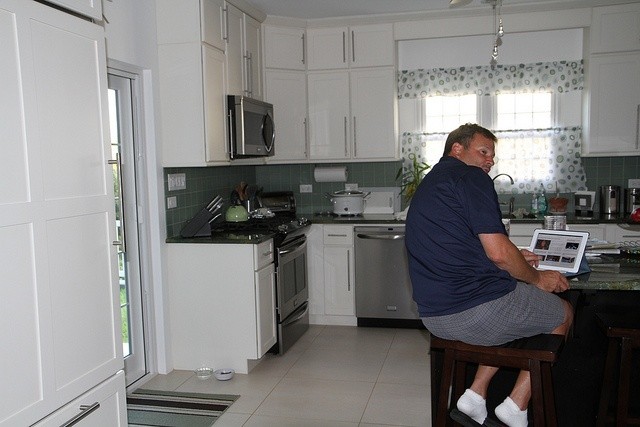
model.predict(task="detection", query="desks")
[517,243,635,426]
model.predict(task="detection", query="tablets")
[529,227,590,273]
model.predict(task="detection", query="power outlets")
[168,173,187,190]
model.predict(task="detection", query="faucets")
[492,173,514,184]
[509,197,515,213]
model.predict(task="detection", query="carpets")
[125,387,240,427]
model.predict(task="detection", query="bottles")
[530,192,538,214]
[538,193,548,214]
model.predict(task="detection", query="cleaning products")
[530,195,538,215]
[538,188,547,215]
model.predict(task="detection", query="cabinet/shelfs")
[2,3,129,426]
[259,69,308,163]
[227,2,261,102]
[581,53,639,158]
[308,23,393,69]
[202,47,228,164]
[583,3,640,54]
[162,238,278,374]
[262,23,308,71]
[323,221,356,326]
[307,71,398,160]
[201,0,229,52]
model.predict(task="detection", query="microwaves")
[227,95,275,160]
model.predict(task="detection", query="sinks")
[502,212,537,219]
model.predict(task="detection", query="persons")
[405,124,575,427]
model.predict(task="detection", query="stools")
[595,311,634,426]
[430,332,565,427]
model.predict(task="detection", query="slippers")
[449,408,502,427]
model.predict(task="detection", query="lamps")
[492,29,497,65]
[498,2,504,36]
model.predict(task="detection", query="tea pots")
[224,191,249,222]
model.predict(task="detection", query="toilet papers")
[314,166,348,181]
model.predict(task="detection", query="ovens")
[275,233,309,356]
[352,225,421,320]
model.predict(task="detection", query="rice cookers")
[324,187,371,217]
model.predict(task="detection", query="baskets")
[617,239,640,263]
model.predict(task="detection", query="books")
[584,245,622,255]
